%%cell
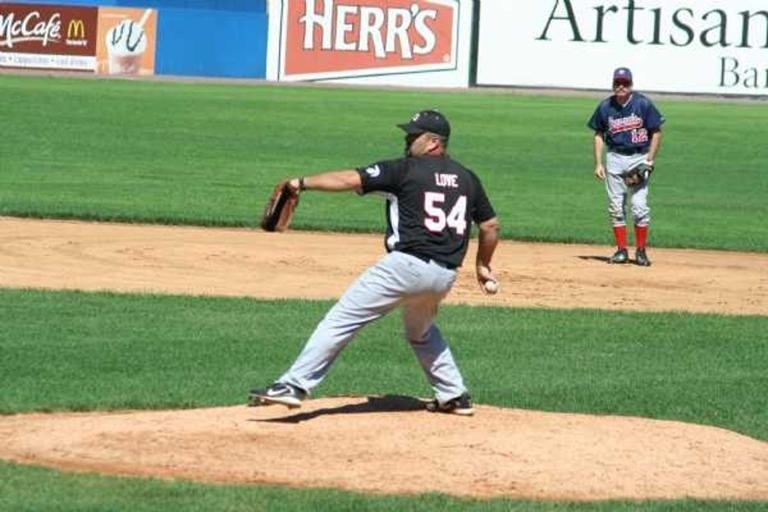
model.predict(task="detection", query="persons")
[585,67,664,266]
[247,109,499,416]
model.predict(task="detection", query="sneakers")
[635,247,651,266]
[608,248,629,263]
[424,391,475,416]
[247,382,307,408]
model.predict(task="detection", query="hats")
[396,110,450,136]
[613,67,632,81]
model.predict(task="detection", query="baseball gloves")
[260,181,299,233]
[623,159,654,187]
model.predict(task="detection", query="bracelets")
[298,177,304,190]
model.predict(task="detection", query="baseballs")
[485,280,499,293]
